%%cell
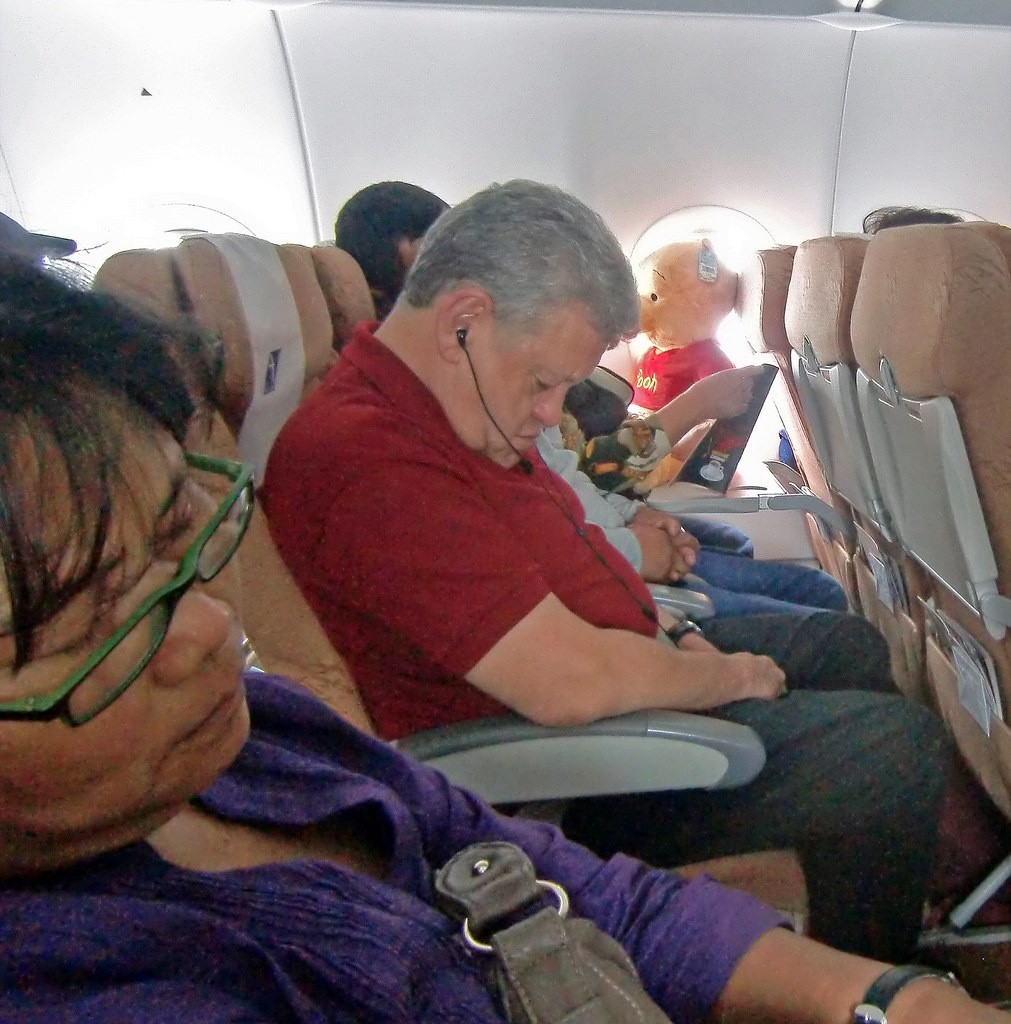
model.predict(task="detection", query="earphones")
[454,325,469,350]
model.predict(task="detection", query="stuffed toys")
[626,241,752,419]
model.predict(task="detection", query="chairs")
[0,222,1010,944]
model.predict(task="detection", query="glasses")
[0,451,256,728]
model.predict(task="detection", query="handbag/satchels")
[436,839,673,1024]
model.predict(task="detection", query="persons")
[0,254,1011,1024]
[258,179,965,963]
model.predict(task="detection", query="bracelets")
[666,620,702,642]
[854,963,971,1024]
[623,417,656,458]
